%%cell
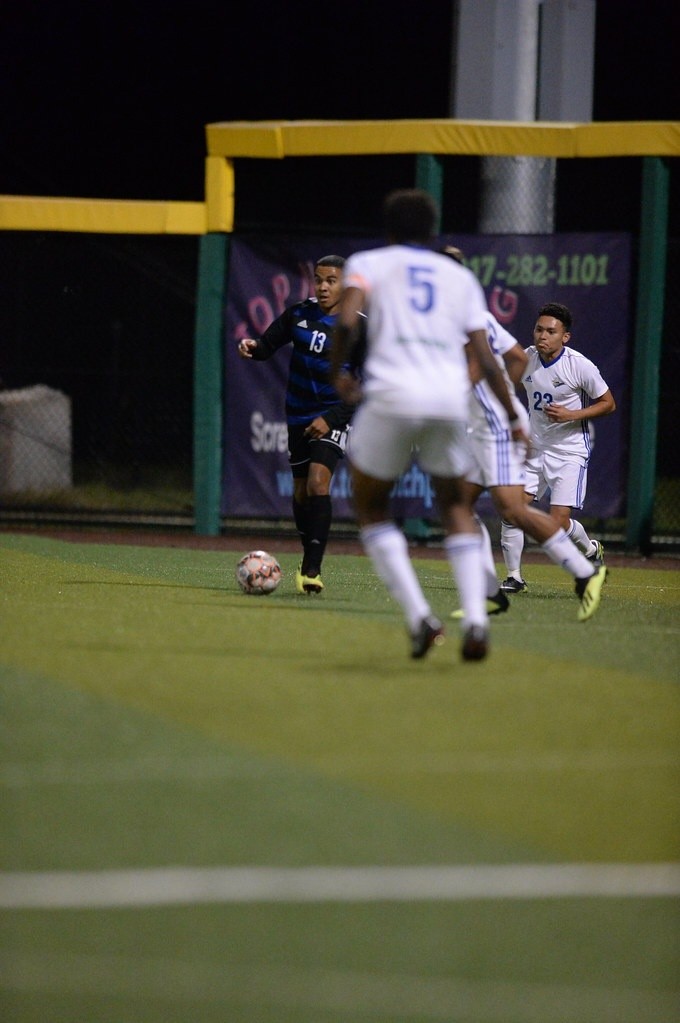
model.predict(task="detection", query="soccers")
[233,551,280,598]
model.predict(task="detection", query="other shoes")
[303,574,323,594]
[462,626,487,661]
[295,560,306,593]
[410,614,443,657]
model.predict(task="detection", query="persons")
[329,191,616,663]
[238,255,369,595]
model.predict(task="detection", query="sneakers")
[583,540,604,568]
[449,589,509,618]
[499,576,528,594]
[574,565,608,622]
